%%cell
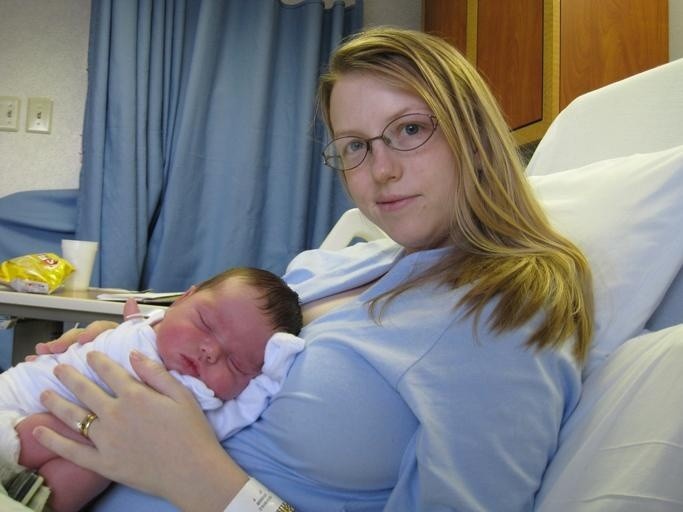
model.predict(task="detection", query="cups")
[61,239,99,291]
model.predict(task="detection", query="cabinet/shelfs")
[422,0,668,149]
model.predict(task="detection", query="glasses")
[322,111,440,170]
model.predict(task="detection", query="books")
[95,291,185,304]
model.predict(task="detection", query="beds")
[319,57,683,512]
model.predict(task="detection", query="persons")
[0,267,304,512]
[24,26,594,512]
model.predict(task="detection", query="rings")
[79,411,95,437]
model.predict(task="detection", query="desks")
[0,286,169,367]
